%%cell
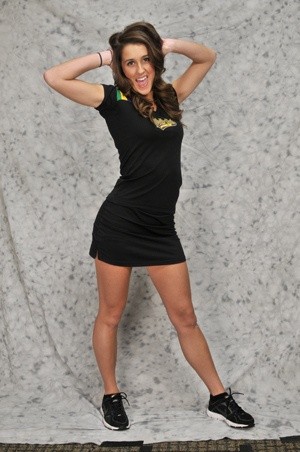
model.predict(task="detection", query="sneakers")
[100,392,131,431]
[207,387,255,429]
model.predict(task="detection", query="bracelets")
[97,52,103,67]
[107,49,113,66]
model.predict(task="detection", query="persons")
[42,20,254,430]
